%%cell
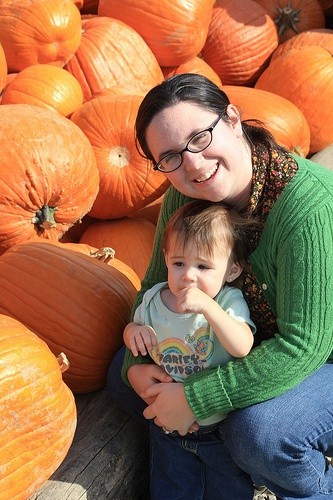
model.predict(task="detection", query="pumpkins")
[0,313,77,500]
[0,243,138,395]
[0,0,333,285]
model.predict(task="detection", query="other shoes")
[253,485,277,500]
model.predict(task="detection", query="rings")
[162,426,173,434]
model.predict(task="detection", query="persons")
[123,197,257,500]
[106,73,333,500]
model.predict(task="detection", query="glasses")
[153,110,226,173]
